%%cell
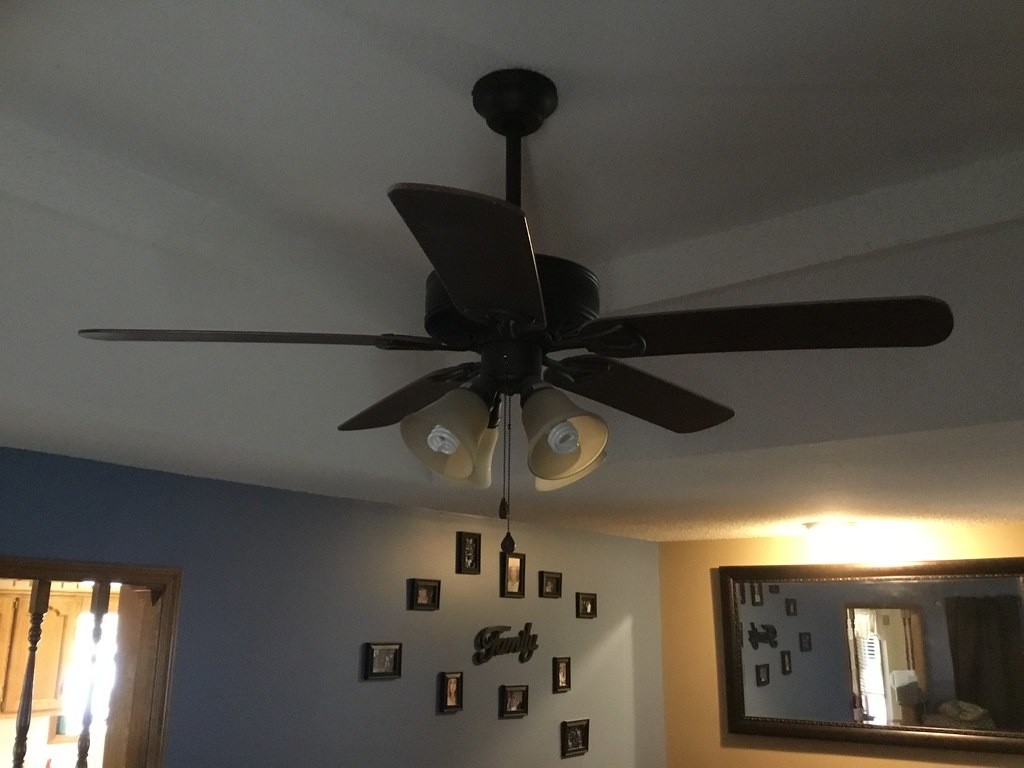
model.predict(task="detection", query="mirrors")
[719,557,1024,755]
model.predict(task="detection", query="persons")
[447,679,457,706]
[507,566,519,592]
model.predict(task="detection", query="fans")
[77,67,954,432]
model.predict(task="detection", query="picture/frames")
[751,584,763,606]
[410,578,440,610]
[756,664,769,685]
[499,685,528,717]
[456,531,480,574]
[553,657,570,692]
[786,599,797,616]
[576,593,596,618]
[562,719,588,756]
[799,632,812,651]
[441,672,462,712]
[769,585,779,593]
[539,571,561,598]
[364,643,401,680]
[782,650,791,675]
[500,552,525,598]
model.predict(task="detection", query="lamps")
[462,392,501,487]
[499,395,509,520]
[534,452,607,492]
[519,379,608,481]
[401,379,496,480]
[500,395,515,554]
